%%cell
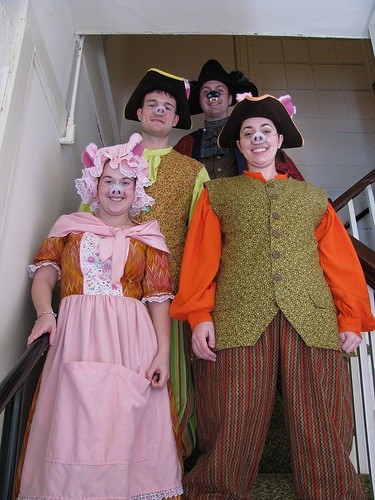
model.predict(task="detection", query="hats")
[74,132,154,217]
[187,59,259,115]
[125,67,193,130]
[218,92,304,148]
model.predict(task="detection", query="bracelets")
[34,312,57,322]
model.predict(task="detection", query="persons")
[168,91,375,500]
[78,68,211,471]
[12,161,183,500]
[173,58,304,181]
